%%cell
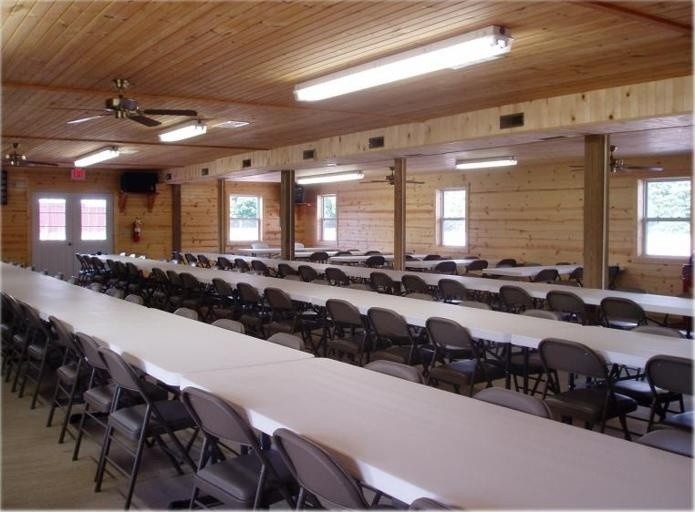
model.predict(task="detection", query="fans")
[0,143,59,168]
[67,79,197,127]
[360,167,426,186]
[570,144,664,174]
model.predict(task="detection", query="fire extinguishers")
[132,218,143,242]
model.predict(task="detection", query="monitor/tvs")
[121,172,156,192]
[295,185,304,203]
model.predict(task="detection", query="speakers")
[0,171,7,204]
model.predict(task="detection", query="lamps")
[295,171,365,184]
[74,145,120,167]
[293,25,512,104]
[158,120,208,143]
[455,155,517,171]
